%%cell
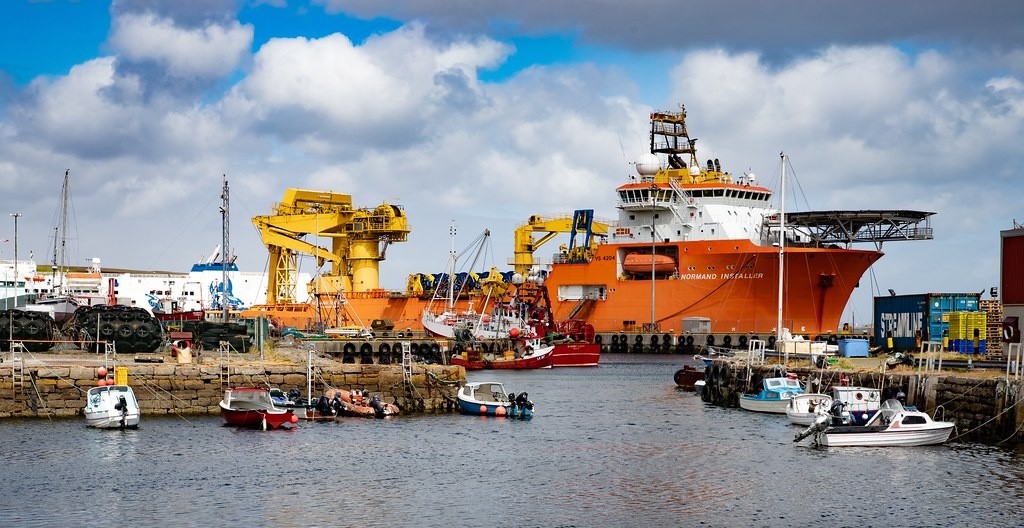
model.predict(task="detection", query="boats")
[784,392,832,427]
[65,273,102,284]
[19,168,85,322]
[831,374,880,426]
[814,400,955,449]
[421,218,532,339]
[218,386,298,431]
[238,103,936,352]
[479,343,557,371]
[264,387,321,421]
[144,288,206,323]
[457,381,536,420]
[450,342,491,370]
[738,376,809,412]
[325,385,401,417]
[25,275,44,281]
[83,384,140,428]
[0,243,313,330]
[519,284,601,368]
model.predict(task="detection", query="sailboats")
[673,150,821,407]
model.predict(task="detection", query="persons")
[750,369,760,394]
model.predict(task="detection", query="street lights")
[9,211,23,309]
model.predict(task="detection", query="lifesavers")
[343,343,534,363]
[1004,325,1013,339]
[173,339,190,350]
[595,334,874,353]
[700,365,746,409]
